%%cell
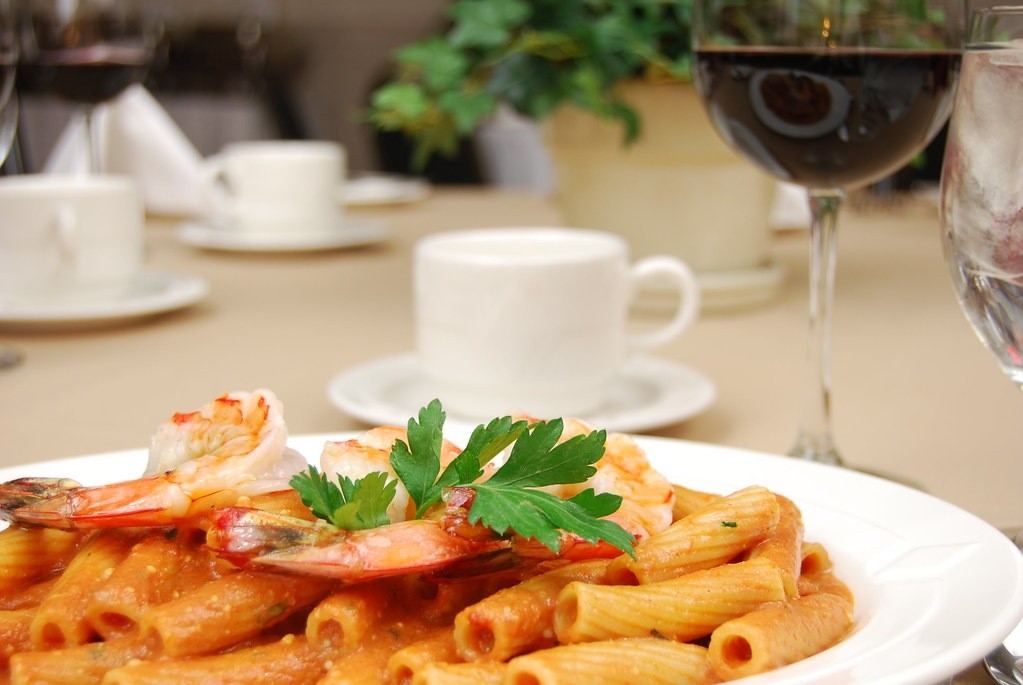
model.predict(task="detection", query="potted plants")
[363,0,1013,290]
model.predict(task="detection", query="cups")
[200,139,346,233]
[0,0,22,176]
[939,6,1023,384]
[0,176,145,302]
[413,226,699,422]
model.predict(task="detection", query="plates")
[1,268,212,329]
[323,348,716,433]
[172,209,391,252]
[0,426,1023,685]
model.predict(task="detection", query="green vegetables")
[290,399,640,561]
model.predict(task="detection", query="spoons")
[984,641,1023,685]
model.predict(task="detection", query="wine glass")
[688,1,966,498]
[22,0,165,175]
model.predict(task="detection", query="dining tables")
[0,186,1023,685]
